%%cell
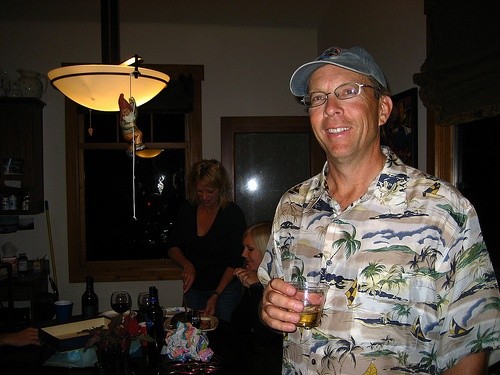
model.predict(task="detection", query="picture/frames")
[380,88,418,168]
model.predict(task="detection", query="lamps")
[47,0,172,112]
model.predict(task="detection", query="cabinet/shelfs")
[0,96,46,216]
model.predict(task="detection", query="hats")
[289,46,387,97]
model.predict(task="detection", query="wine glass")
[110,291,132,328]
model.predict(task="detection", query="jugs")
[16,68,48,98]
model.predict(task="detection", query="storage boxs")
[39,315,112,352]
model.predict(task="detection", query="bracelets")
[211,290,221,298]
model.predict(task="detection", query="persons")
[159,158,248,363]
[216,221,273,375]
[257,45,500,375]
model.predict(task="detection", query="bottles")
[143,287,167,370]
[6,80,22,97]
[81,276,99,320]
[2,191,33,212]
[17,252,42,273]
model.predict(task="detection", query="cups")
[282,281,330,328]
[54,301,73,323]
[137,291,150,311]
[139,321,147,347]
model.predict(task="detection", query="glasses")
[300,81,383,109]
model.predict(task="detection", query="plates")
[164,315,218,331]
[163,307,192,317]
